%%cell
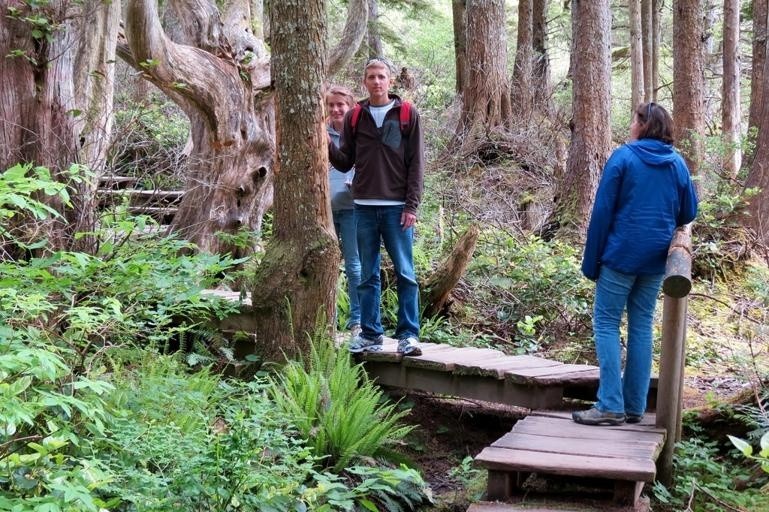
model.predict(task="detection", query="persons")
[572,102,698,426]
[325,85,363,343]
[327,61,422,356]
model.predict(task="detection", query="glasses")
[647,102,656,117]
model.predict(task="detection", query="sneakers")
[396,337,423,356]
[346,322,385,353]
[625,413,644,425]
[572,407,626,427]
